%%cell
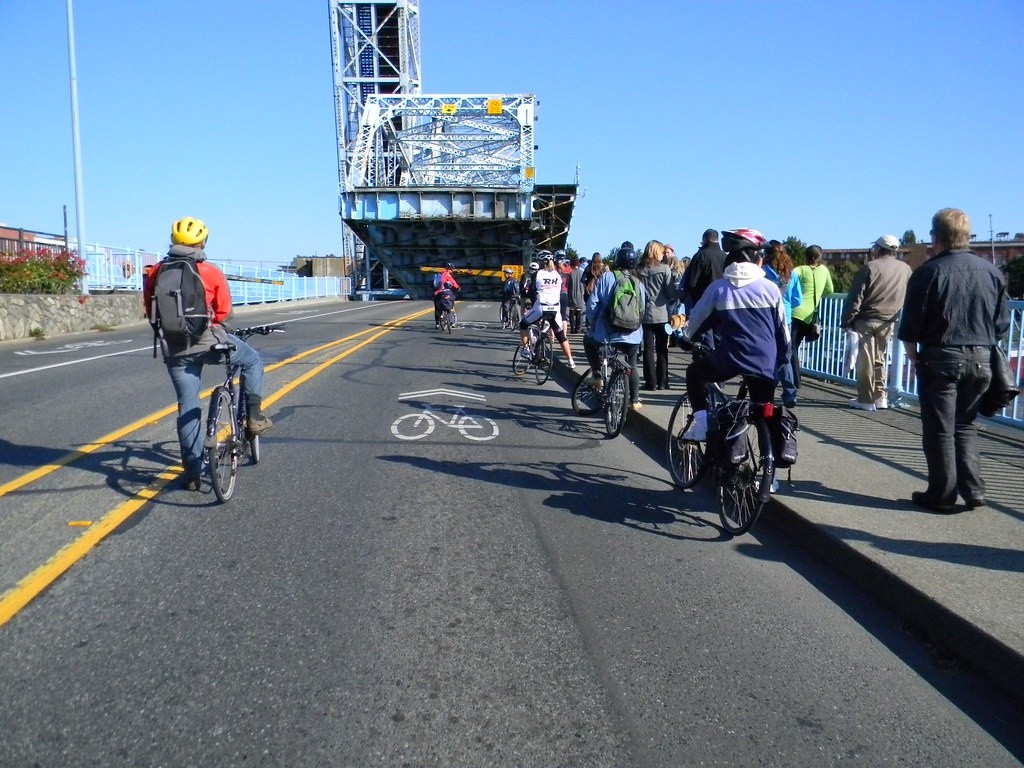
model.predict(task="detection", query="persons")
[791,245,835,390]
[143,217,272,490]
[841,235,912,412]
[520,250,576,370]
[898,208,1010,509]
[501,269,520,326]
[583,248,650,410]
[521,229,795,391]
[434,264,460,327]
[681,230,790,493]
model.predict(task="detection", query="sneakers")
[247,415,273,434]
[181,473,200,491]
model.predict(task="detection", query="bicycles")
[439,307,458,334]
[570,338,630,439]
[499,294,520,331]
[665,342,774,536]
[512,316,554,385]
[202,324,286,504]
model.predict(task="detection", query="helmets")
[536,250,553,262]
[170,216,209,245]
[528,262,539,270]
[446,263,455,269]
[554,249,566,262]
[720,227,773,252]
[444,281,451,289]
[503,269,512,274]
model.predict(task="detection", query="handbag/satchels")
[977,339,1019,417]
[804,310,820,342]
[701,399,799,468]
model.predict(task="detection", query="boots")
[569,309,575,334]
[575,309,582,334]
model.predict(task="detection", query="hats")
[664,244,674,252]
[616,248,636,269]
[870,235,900,251]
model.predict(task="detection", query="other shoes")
[584,376,780,494]
[434,325,439,330]
[965,495,986,508]
[912,490,953,514]
[849,398,876,412]
[875,396,888,409]
[521,349,530,358]
[569,363,577,369]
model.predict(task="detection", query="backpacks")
[603,268,643,331]
[148,254,207,353]
[433,273,442,290]
